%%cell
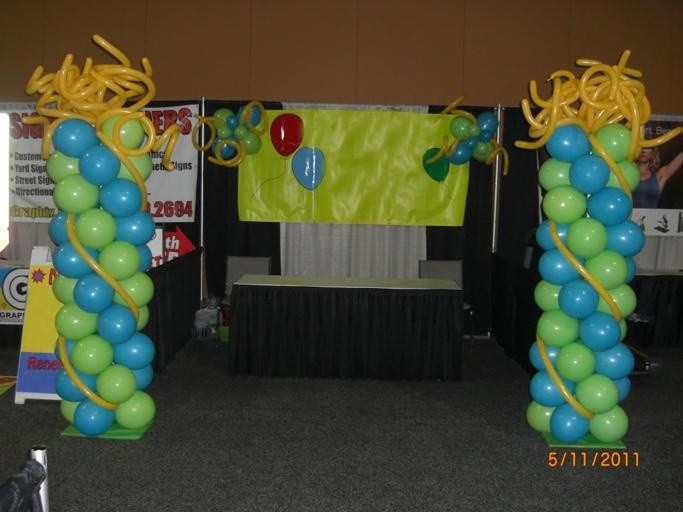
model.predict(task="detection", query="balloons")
[421,95,509,183]
[19,33,179,436]
[191,98,326,191]
[515,49,682,442]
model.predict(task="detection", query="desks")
[230,275,462,386]
[634,268,682,349]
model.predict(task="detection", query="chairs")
[214,256,272,352]
[418,260,476,351]
